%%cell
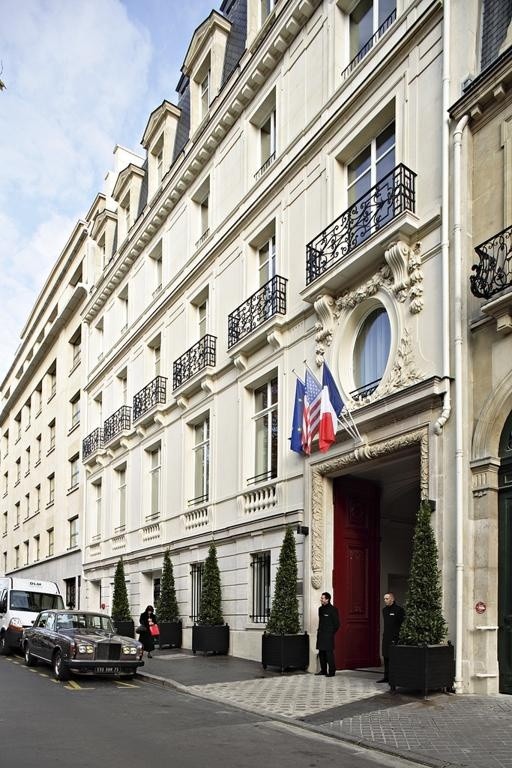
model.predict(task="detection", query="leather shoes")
[314,671,338,677]
[376,678,388,683]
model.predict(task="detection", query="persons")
[376,592,405,686]
[138,606,157,658]
[315,593,338,677]
[98,604,107,613]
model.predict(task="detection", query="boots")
[147,654,152,658]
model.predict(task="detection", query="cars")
[20,609,144,680]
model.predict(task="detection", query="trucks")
[0,576,66,654]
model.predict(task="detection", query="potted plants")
[383,496,457,701]
[261,526,310,674]
[153,539,231,656]
[109,554,136,639]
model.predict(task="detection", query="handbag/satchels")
[149,624,161,637]
[135,625,146,634]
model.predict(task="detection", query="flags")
[290,362,344,456]
